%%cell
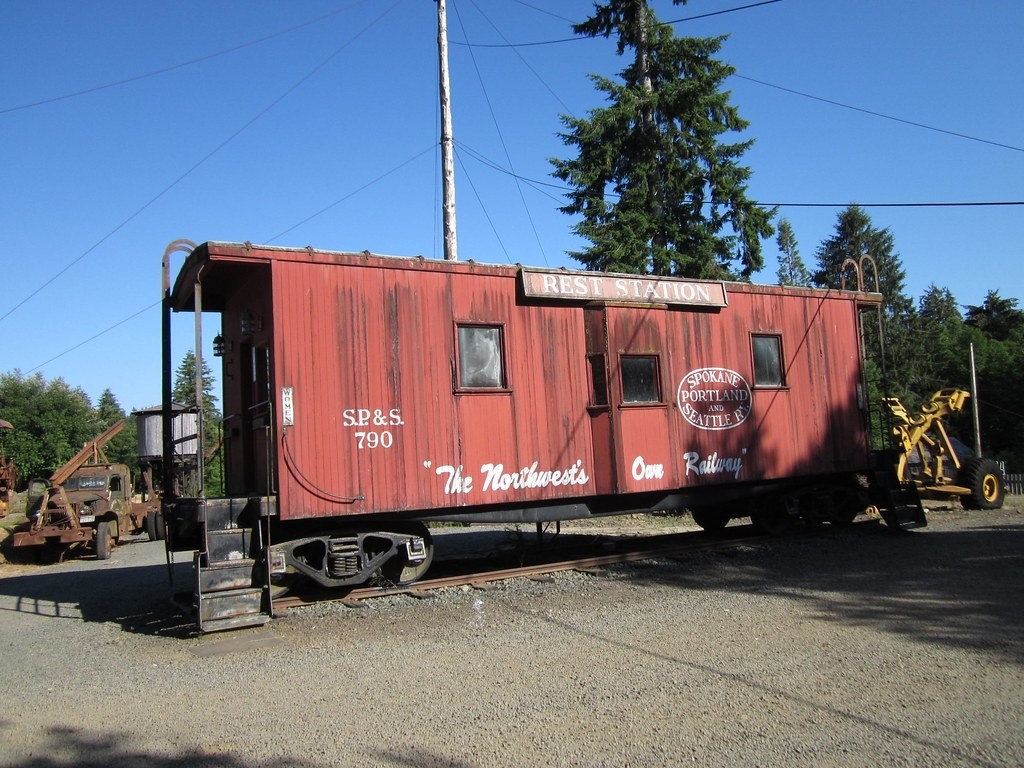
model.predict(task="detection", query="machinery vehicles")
[0,418,16,517]
[879,387,1008,511]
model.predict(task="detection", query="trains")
[160,239,927,633]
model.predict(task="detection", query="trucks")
[12,418,159,560]
